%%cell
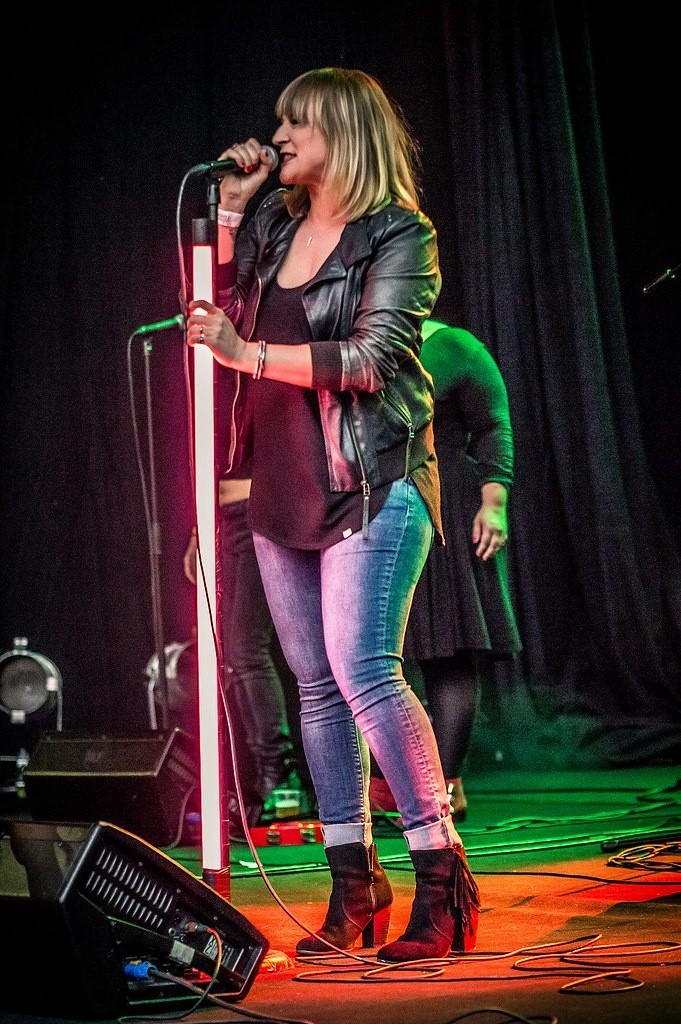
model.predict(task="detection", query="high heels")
[295,841,393,956]
[376,847,481,963]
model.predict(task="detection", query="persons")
[180,67,480,964]
[369,316,520,826]
[179,337,309,844]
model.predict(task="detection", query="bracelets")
[254,340,266,381]
[216,207,243,229]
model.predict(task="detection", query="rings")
[498,540,504,546]
[200,334,204,344]
[200,326,203,333]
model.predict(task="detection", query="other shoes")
[368,776,468,835]
[227,824,252,843]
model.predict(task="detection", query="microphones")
[135,313,184,335]
[190,145,279,178]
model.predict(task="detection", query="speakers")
[22,727,202,848]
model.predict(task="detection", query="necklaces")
[307,212,336,245]
